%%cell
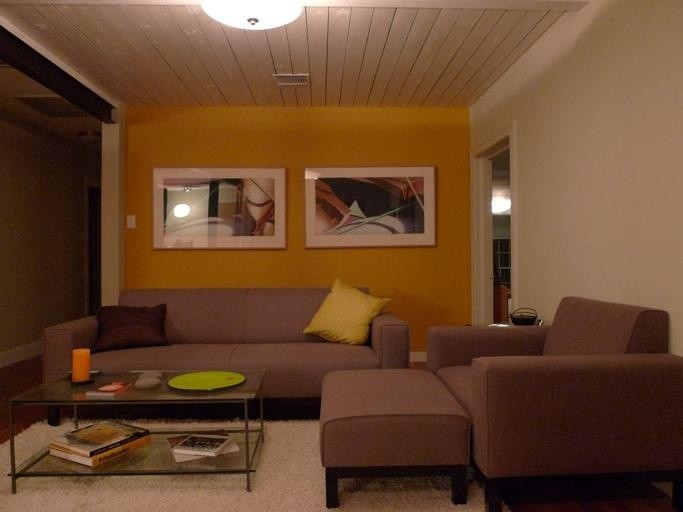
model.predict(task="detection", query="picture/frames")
[150,165,437,251]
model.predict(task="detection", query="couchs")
[39,287,411,426]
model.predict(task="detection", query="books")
[45,432,150,468]
[85,380,132,397]
[172,432,232,457]
[48,418,149,457]
[164,428,239,462]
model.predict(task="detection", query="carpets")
[0,417,512,511]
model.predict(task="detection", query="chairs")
[423,296,682,512]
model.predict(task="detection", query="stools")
[319,368,473,507]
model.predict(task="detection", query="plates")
[167,370,245,392]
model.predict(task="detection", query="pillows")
[301,277,392,345]
[91,303,170,353]
